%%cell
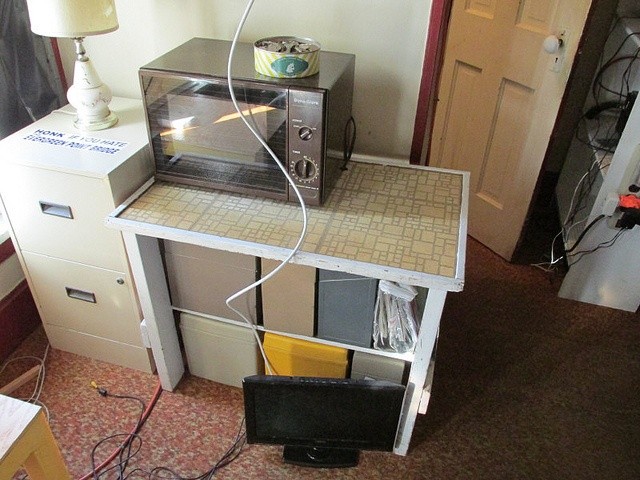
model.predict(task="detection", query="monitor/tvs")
[242,374,406,468]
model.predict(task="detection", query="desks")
[106,155,470,457]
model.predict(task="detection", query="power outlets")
[607,144,640,231]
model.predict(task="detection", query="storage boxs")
[262,331,348,381]
[178,313,261,388]
[163,239,261,325]
[318,268,378,348]
[261,258,319,337]
[351,351,403,385]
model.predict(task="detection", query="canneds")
[253,36,321,79]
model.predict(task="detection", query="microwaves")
[136,37,356,209]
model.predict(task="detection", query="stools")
[0,395,73,480]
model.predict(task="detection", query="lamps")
[26,0,119,132]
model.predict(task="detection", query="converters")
[620,207,638,230]
[599,192,620,217]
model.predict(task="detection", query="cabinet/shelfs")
[556,3,640,314]
[0,95,156,375]
